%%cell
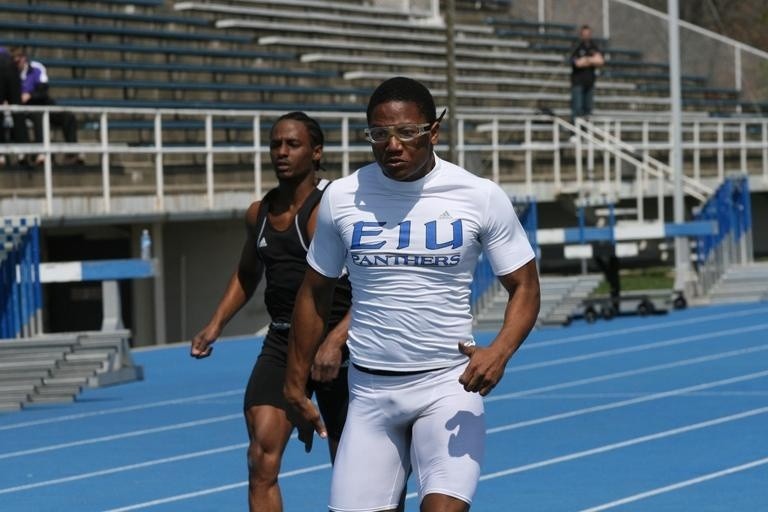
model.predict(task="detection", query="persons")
[279,75,542,511]
[10,48,85,165]
[188,112,353,511]
[565,24,606,143]
[0,50,35,166]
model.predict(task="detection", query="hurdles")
[693,173,766,305]
[469,196,605,329]
[0,215,133,411]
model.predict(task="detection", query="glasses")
[364,122,431,146]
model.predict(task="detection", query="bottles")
[140,228,153,261]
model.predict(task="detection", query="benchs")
[0,0,767,174]
